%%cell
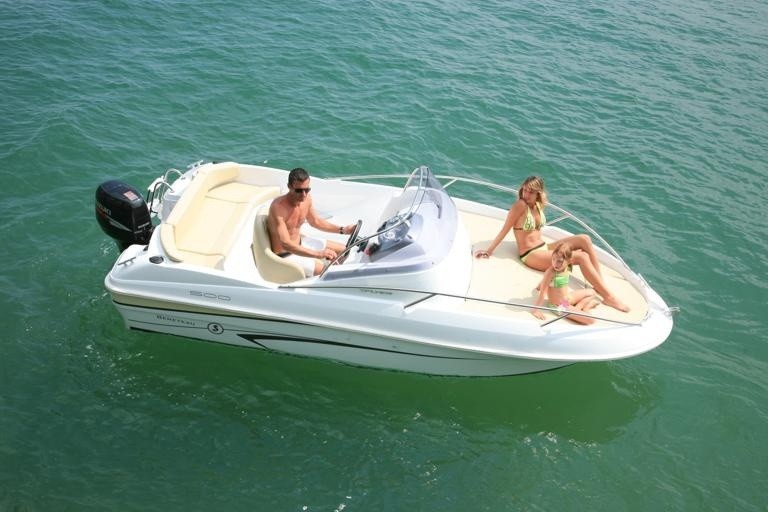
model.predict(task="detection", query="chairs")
[253,197,307,282]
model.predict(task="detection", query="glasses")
[293,187,311,192]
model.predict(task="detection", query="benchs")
[160,162,278,272]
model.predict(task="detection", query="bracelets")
[339,226,344,235]
[482,251,488,258]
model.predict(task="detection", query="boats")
[95,160,673,376]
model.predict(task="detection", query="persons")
[531,243,595,326]
[269,168,357,278]
[475,175,630,312]
